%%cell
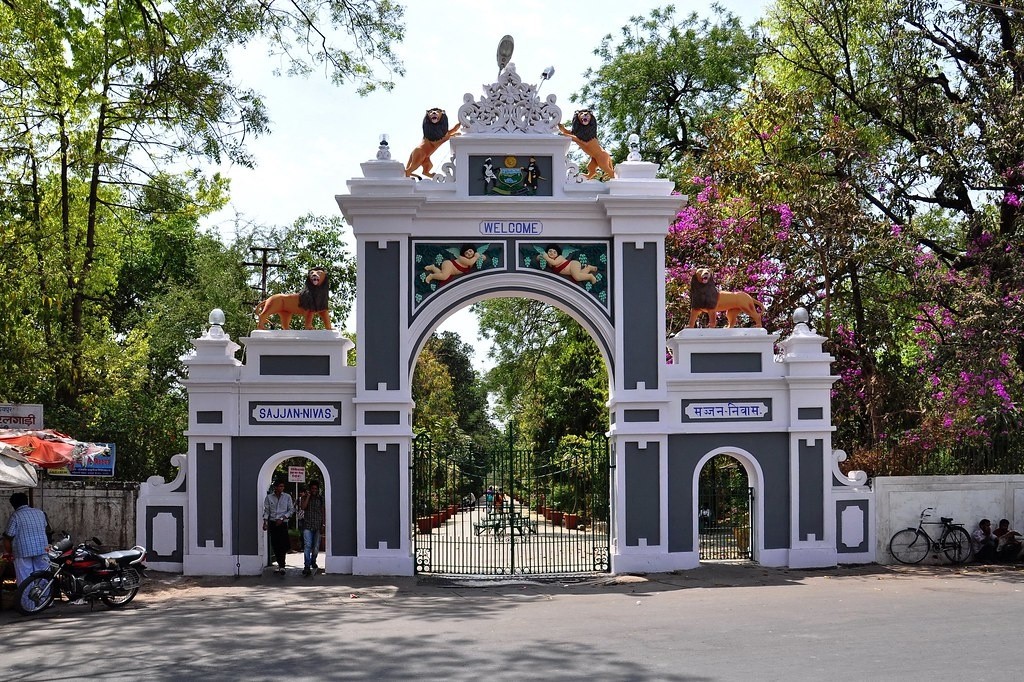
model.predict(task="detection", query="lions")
[404,107,462,182]
[255,266,336,330]
[686,267,765,328]
[557,110,614,182]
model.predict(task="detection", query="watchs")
[280,517,284,520]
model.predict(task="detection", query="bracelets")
[323,524,326,527]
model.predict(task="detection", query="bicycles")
[890,507,972,565]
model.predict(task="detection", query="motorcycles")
[16,530,148,616]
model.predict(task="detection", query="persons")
[469,486,505,513]
[970,519,1024,564]
[263,480,325,575]
[424,244,486,283]
[5,491,53,613]
[520,157,541,190]
[537,244,597,284]
[482,158,499,194]
[699,505,712,527]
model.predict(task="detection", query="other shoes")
[310,562,317,569]
[301,565,311,578]
[272,566,286,576]
